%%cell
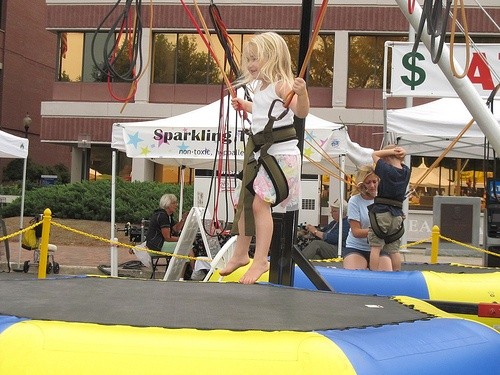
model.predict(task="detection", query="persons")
[217,31,310,285]
[342,166,393,271]
[300,198,350,261]
[367,144,411,272]
[145,193,196,280]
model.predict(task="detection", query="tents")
[110,86,348,280]
[0,130,29,269]
[384,98,500,173]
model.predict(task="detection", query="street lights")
[22,111,32,180]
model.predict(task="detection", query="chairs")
[141,218,172,279]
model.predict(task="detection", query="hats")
[328,198,348,210]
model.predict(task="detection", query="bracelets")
[314,230,318,236]
